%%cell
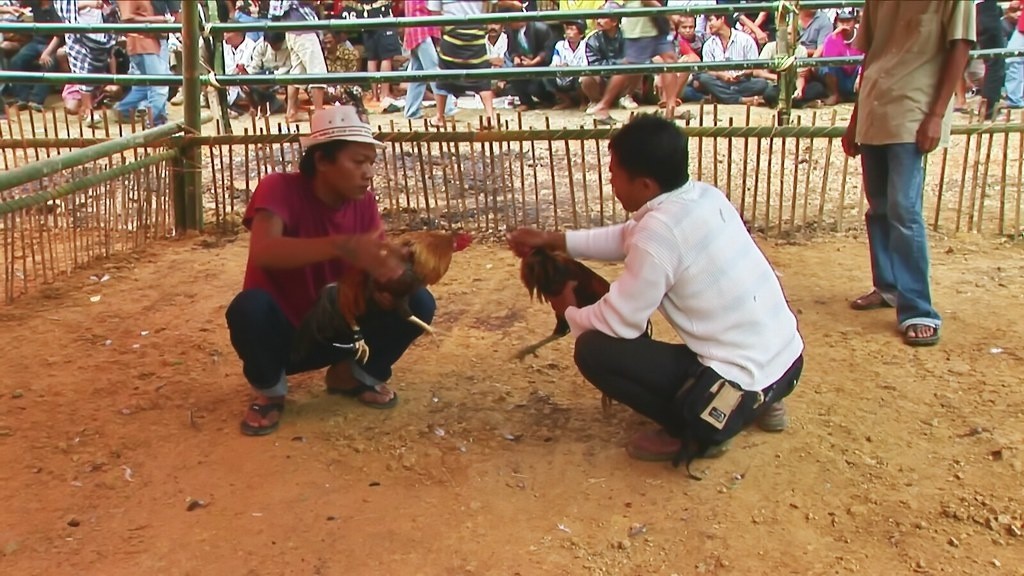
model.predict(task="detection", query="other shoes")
[170,88,188,105]
[0,105,11,120]
[954,108,980,117]
[15,100,29,110]
[585,102,620,126]
[28,100,44,112]
[199,92,211,107]
[626,427,725,459]
[85,111,105,126]
[677,110,698,119]
[756,400,786,432]
[618,95,639,109]
[113,111,148,124]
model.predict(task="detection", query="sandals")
[904,322,941,347]
[850,290,892,310]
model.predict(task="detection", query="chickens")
[335,228,473,366]
[505,223,653,414]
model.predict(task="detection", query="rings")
[380,249,388,258]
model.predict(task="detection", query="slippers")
[473,125,500,131]
[240,400,283,436]
[326,381,399,409]
[426,119,445,128]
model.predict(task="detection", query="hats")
[836,7,857,20]
[299,105,387,150]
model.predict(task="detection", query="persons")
[225,106,436,437]
[0,0,867,128]
[507,112,805,460]
[842,0,977,347]
[951,0,1024,121]
